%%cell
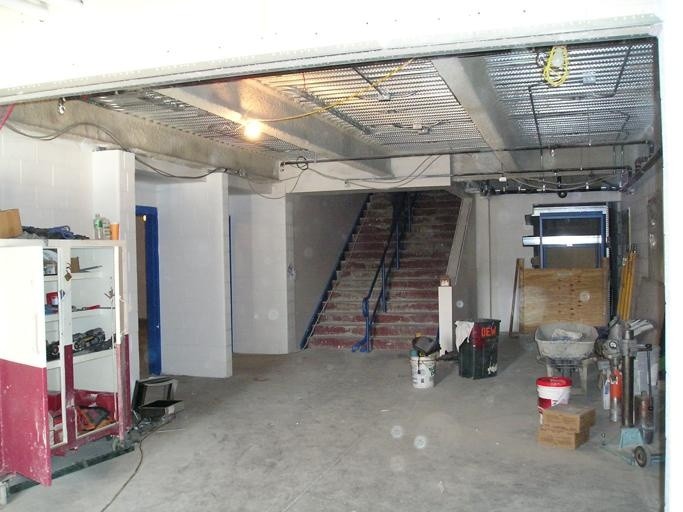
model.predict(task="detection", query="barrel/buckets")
[536,376,572,425]
[409,355,437,389]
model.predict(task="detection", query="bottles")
[93,213,110,240]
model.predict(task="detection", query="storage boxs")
[538,402,596,450]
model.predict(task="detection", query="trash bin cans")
[458,318,501,379]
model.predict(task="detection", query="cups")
[110,223,119,240]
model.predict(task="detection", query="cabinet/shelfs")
[0,240,131,486]
